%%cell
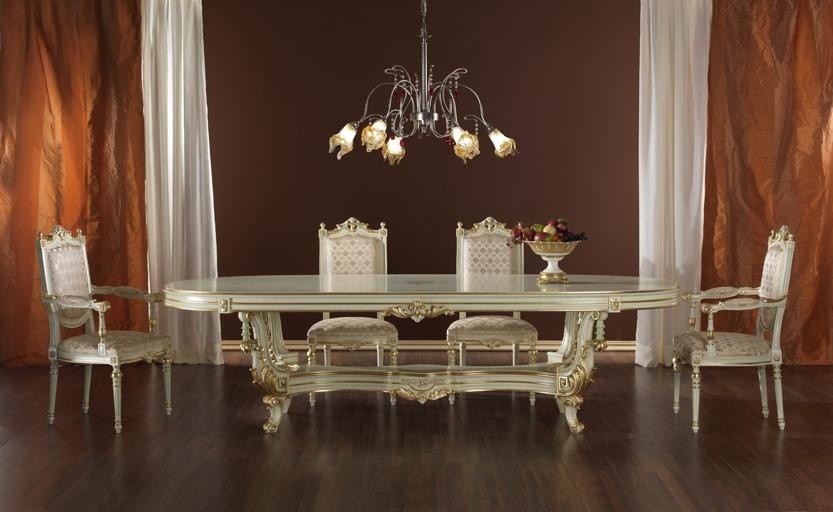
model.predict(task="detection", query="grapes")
[511,221,532,244]
[561,231,588,242]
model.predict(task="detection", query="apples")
[521,218,570,242]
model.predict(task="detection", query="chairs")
[307,218,398,406]
[38,224,173,435]
[446,217,538,406]
[673,224,798,434]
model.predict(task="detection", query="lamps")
[328,0,518,167]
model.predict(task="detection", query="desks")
[162,272,679,434]
